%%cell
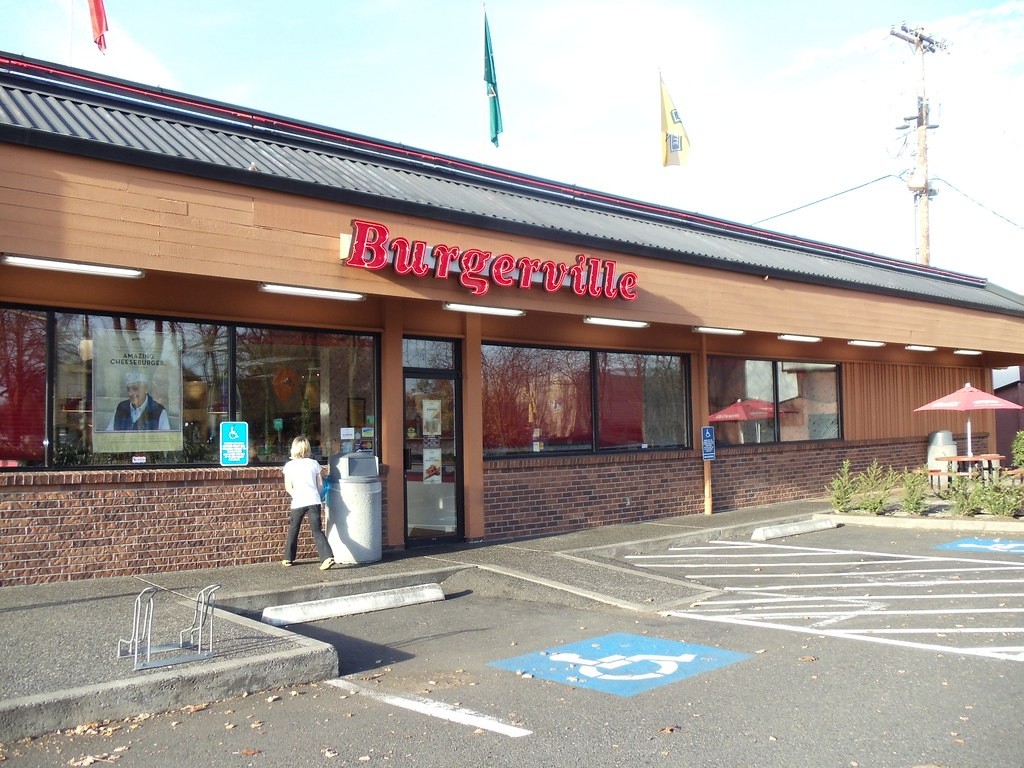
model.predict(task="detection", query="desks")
[935,456,1005,496]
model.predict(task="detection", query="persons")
[106,371,171,430]
[282,436,336,570]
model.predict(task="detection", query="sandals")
[320,558,335,570]
[282,560,292,567]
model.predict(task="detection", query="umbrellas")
[913,382,1024,471]
[744,397,799,443]
[708,398,770,444]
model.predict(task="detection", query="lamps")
[78,315,103,361]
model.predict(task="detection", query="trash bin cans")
[927,429,958,484]
[322,452,383,565]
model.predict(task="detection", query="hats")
[125,371,147,384]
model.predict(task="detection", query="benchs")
[913,469,1024,501]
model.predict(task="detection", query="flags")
[87,0,108,55]
[660,79,691,166]
[483,14,503,148]
